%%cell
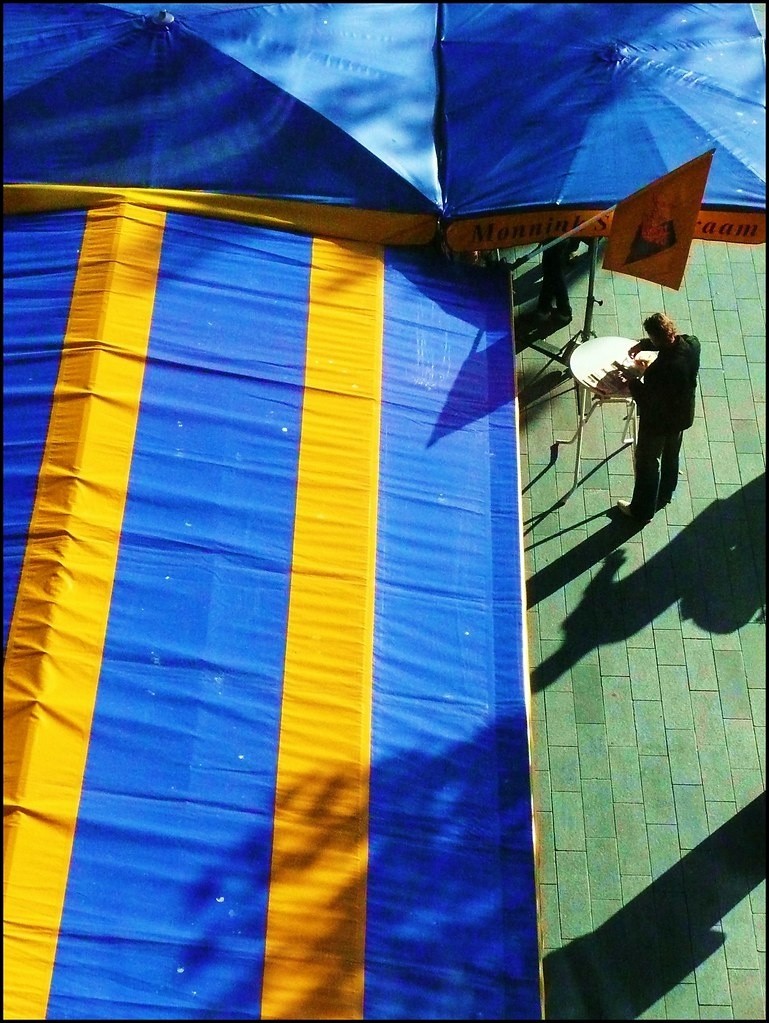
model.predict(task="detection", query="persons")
[617,312,701,521]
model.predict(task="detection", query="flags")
[601,152,712,290]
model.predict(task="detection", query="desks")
[556,337,660,491]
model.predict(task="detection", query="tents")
[0,185,545,1022]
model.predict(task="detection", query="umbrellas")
[0,0,445,247]
[440,0,769,345]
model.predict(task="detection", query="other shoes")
[617,500,653,523]
